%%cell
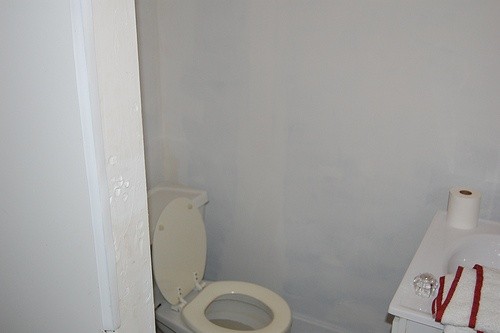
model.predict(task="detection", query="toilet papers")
[446,187,482,230]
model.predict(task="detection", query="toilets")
[147,185,293,333]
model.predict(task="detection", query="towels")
[433,267,500,333]
